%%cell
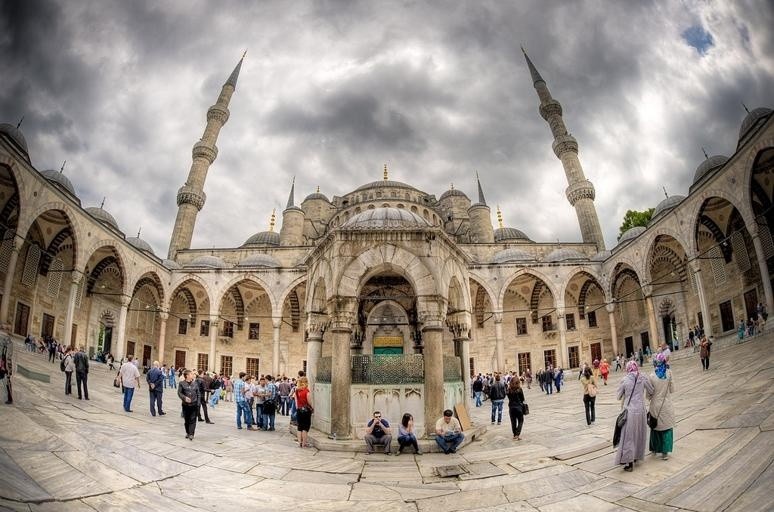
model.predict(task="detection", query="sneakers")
[299,442,309,447]
[624,464,634,472]
[659,453,668,461]
[528,386,562,394]
[491,420,502,426]
[412,448,422,454]
[236,422,275,432]
[385,451,393,456]
[184,433,195,440]
[65,390,90,402]
[395,450,401,457]
[365,450,375,455]
[513,435,522,442]
[651,451,658,457]
[124,407,166,416]
[445,448,457,454]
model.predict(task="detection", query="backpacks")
[60,358,66,373]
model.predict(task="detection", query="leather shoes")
[206,421,215,424]
[198,418,205,422]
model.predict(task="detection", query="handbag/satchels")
[587,381,599,397]
[523,403,530,416]
[647,412,658,430]
[616,409,628,428]
[262,399,277,416]
[113,375,121,388]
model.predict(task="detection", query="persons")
[435,409,464,453]
[644,349,678,459]
[395,413,423,456]
[616,358,654,472]
[364,411,393,455]
[0,320,15,404]
[23,335,314,448]
